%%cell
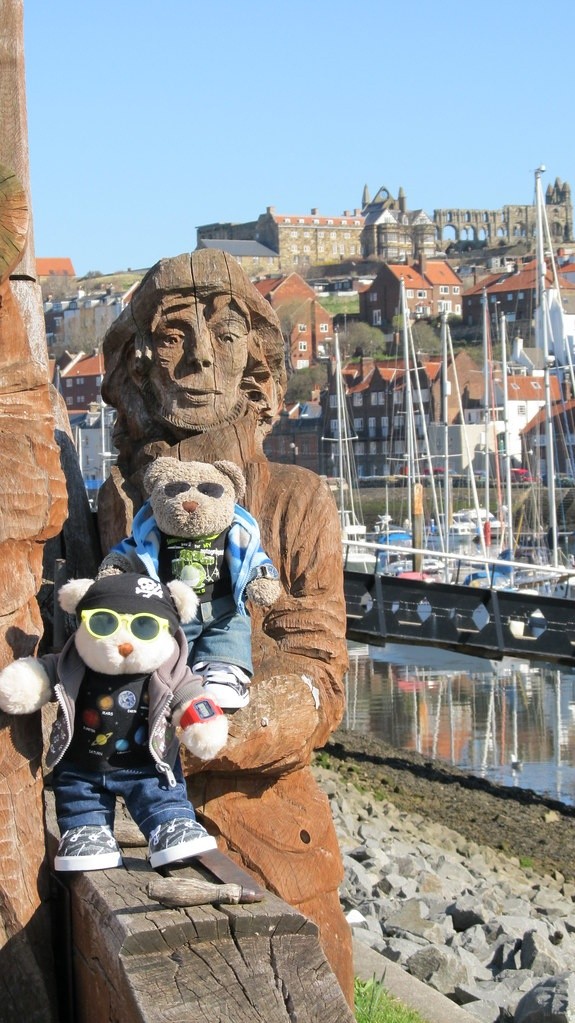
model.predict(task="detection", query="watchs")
[247,564,280,581]
[180,697,223,732]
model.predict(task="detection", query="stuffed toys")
[101,455,286,710]
[1,576,231,872]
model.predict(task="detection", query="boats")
[324,166,575,667]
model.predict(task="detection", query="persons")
[97,249,348,1008]
